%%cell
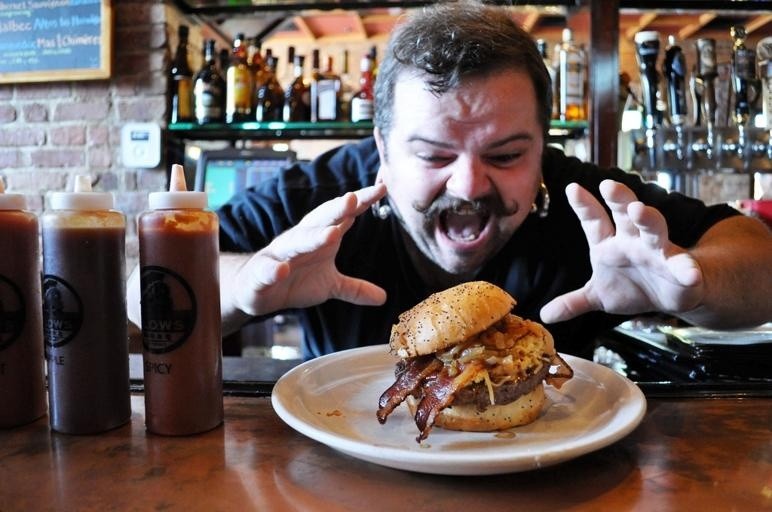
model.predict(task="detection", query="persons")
[126,0,772,355]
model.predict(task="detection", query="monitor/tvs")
[194,150,295,212]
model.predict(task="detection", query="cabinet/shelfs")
[163,0,772,208]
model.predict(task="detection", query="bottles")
[217,48,228,78]
[42,175,132,435]
[255,58,284,124]
[318,56,339,121]
[193,60,226,125]
[559,28,589,122]
[244,40,261,69]
[262,49,274,67]
[0,176,48,435]
[536,38,558,119]
[137,164,224,437]
[336,50,356,117]
[286,46,296,81]
[310,49,323,122]
[367,45,378,79]
[347,55,374,122]
[202,39,214,69]
[169,25,191,123]
[226,33,252,124]
[282,56,311,122]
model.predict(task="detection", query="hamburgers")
[376,281,574,444]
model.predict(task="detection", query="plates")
[270,341,649,479]
